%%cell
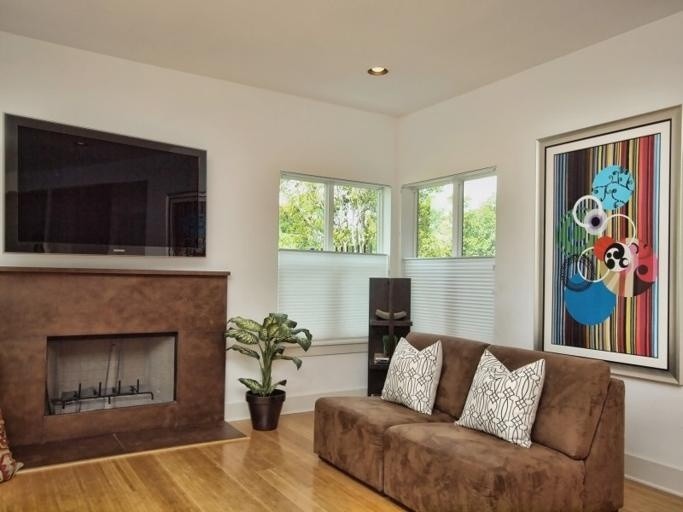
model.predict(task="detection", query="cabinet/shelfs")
[367,276,412,395]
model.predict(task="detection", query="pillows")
[377,336,545,450]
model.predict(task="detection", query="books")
[373,358,390,364]
[373,351,389,361]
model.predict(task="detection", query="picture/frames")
[533,105,682,385]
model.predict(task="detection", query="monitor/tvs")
[3,113,207,256]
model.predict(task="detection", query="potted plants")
[222,310,312,430]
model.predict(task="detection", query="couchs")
[315,332,624,512]
